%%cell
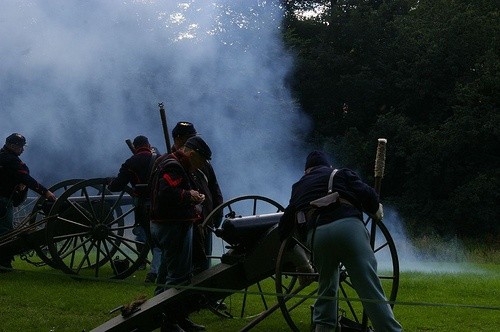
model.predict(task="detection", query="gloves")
[376,202,384,220]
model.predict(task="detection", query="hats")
[186,135,212,161]
[305,149,331,171]
[172,121,197,138]
[5,133,29,147]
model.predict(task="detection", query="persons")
[0,133,56,269]
[154,122,228,310]
[145,136,212,296]
[110,135,162,272]
[277,150,403,332]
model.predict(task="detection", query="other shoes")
[153,287,164,296]
[178,319,206,332]
[210,301,234,320]
[144,272,157,283]
